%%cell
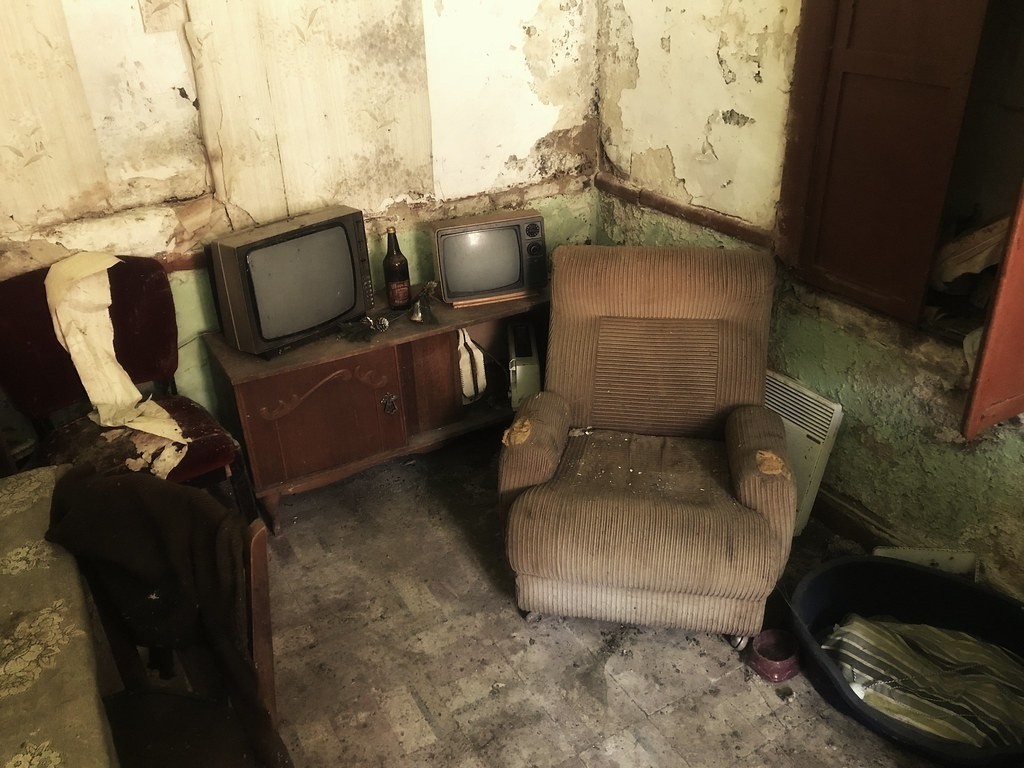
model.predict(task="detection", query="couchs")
[498,245,798,638]
[0,250,277,712]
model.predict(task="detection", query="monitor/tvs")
[208,202,374,362]
[429,208,547,308]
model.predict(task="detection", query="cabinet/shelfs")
[199,282,556,539]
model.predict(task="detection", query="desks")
[0,458,124,767]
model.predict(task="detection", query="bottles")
[384,227,412,310]
